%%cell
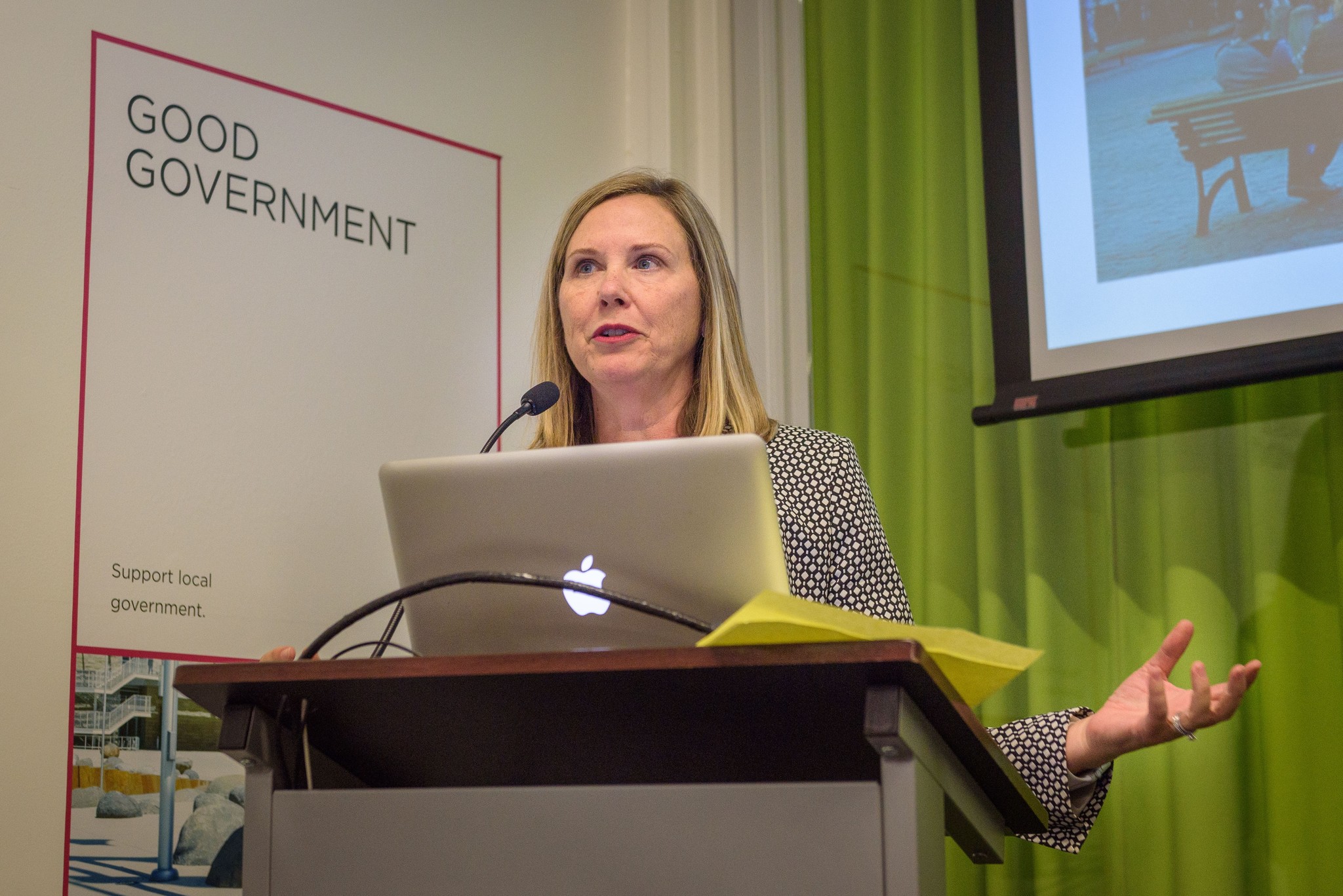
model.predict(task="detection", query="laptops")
[379,434,791,658]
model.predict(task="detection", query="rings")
[1171,714,1196,742]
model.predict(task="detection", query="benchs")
[1147,75,1342,237]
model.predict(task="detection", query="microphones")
[372,383,561,657]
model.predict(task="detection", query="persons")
[258,172,1261,857]
[1212,0,1343,198]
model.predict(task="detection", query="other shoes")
[1286,174,1343,199]
